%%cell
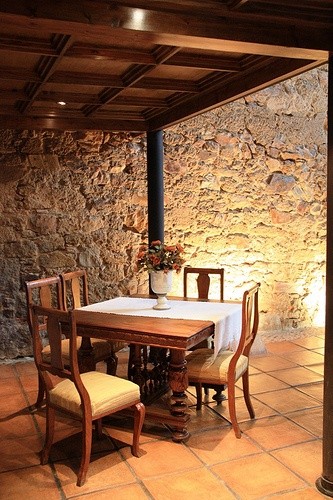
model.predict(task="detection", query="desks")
[61,295,241,444]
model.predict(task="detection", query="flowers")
[134,240,187,274]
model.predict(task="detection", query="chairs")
[27,270,146,487]
[170,265,225,395]
[188,282,268,440]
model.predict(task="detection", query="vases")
[151,270,176,311]
